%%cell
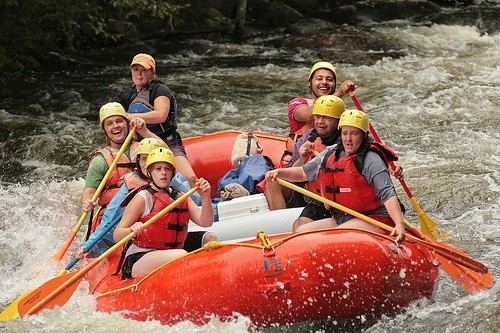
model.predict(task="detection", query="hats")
[128,53,155,72]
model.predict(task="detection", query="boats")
[79,130,440,326]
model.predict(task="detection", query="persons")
[281,61,358,168]
[266,95,404,211]
[265,109,408,242]
[113,147,217,278]
[81,102,160,213]
[77,138,200,260]
[126,53,197,189]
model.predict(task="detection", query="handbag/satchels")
[221,183,250,201]
[232,133,263,168]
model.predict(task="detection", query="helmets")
[100,102,130,127]
[307,62,336,82]
[312,95,345,118]
[337,109,370,132]
[146,147,176,168]
[136,137,168,155]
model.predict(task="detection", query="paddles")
[352,85,450,243]
[53,123,137,262]
[268,174,489,276]
[405,212,480,291]
[18,174,201,318]
[0,211,123,323]
